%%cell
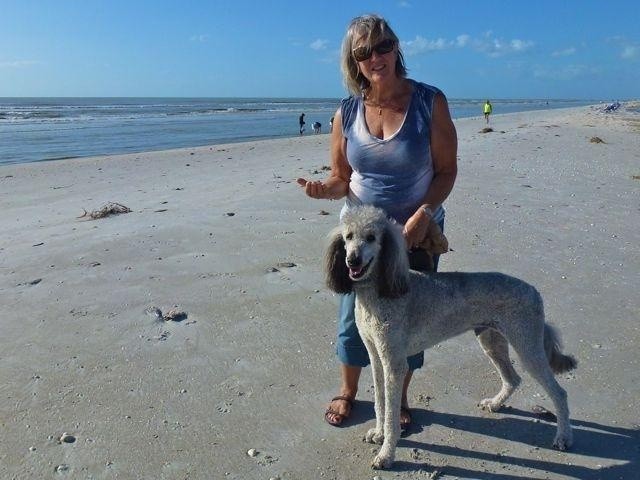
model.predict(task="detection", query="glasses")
[354,39,394,62]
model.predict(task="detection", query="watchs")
[420,206,434,218]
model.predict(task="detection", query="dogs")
[323,202,578,471]
[299,128,306,136]
[311,122,321,135]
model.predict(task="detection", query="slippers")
[400,405,412,427]
[325,395,353,426]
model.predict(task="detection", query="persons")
[299,113,306,134]
[312,121,321,133]
[329,116,334,132]
[297,14,458,429]
[482,99,492,124]
